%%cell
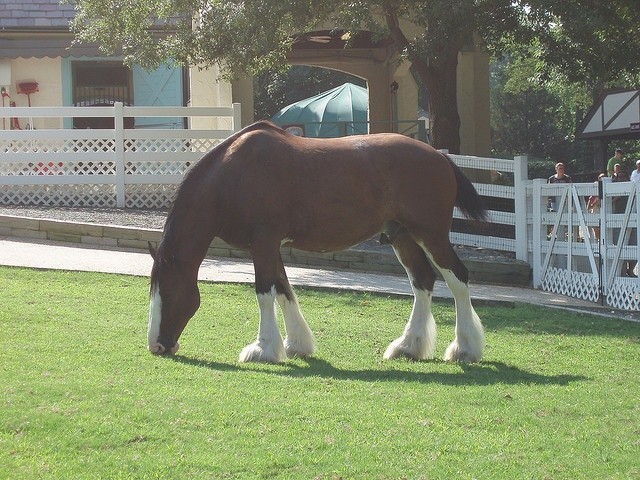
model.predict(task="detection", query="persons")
[586,173,608,240]
[548,163,571,213]
[630,160,640,181]
[606,150,623,177]
[611,163,621,179]
[612,171,638,278]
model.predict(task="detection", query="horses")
[147,120,490,364]
